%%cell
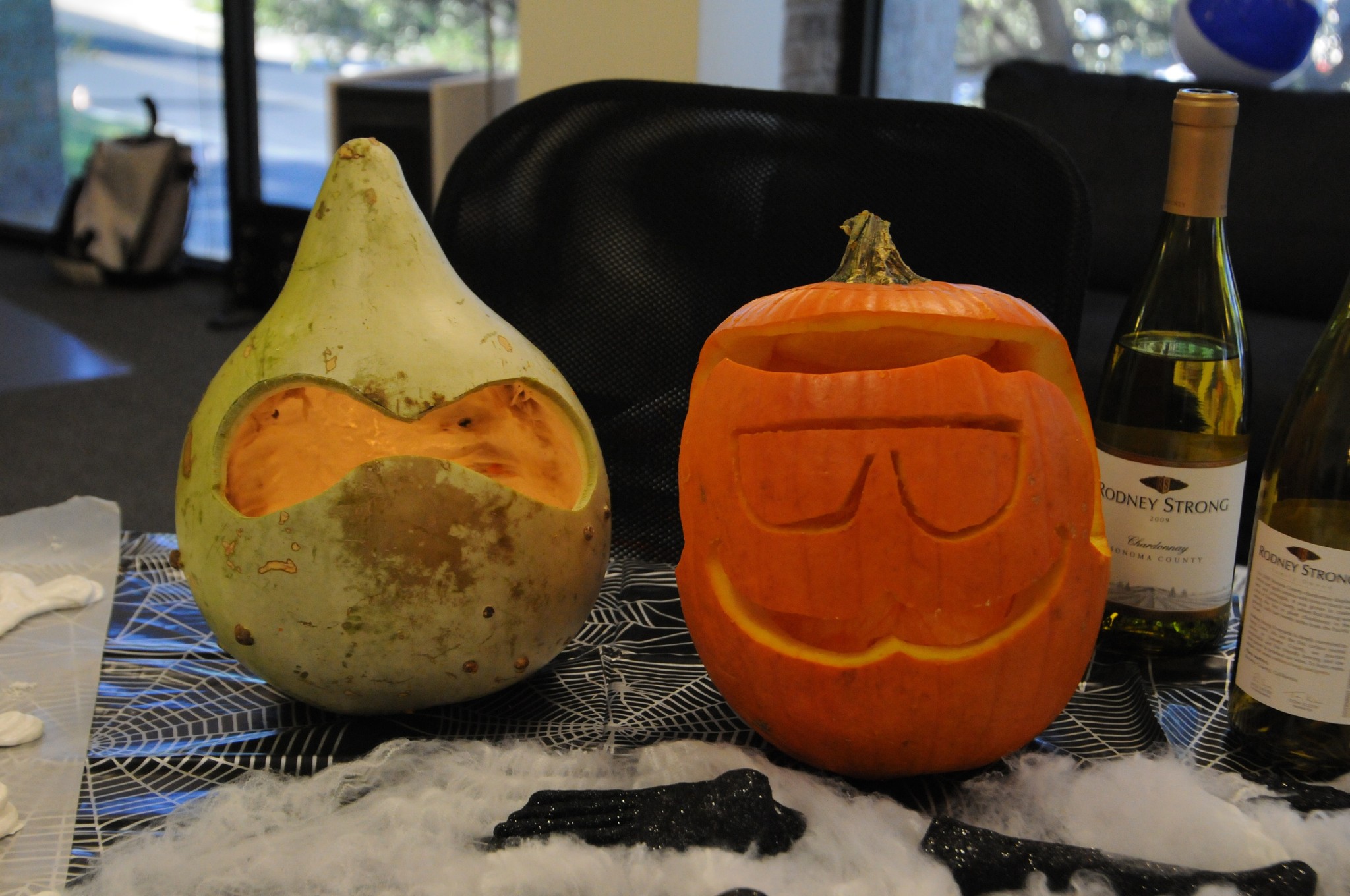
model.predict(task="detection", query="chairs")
[983,55,1350,467]
[431,75,1091,541]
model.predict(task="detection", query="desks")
[63,537,1350,896]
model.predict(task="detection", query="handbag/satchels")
[50,137,201,291]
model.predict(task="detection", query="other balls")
[1170,0,1323,91]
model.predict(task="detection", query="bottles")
[1092,88,1254,677]
[1228,276,1350,778]
